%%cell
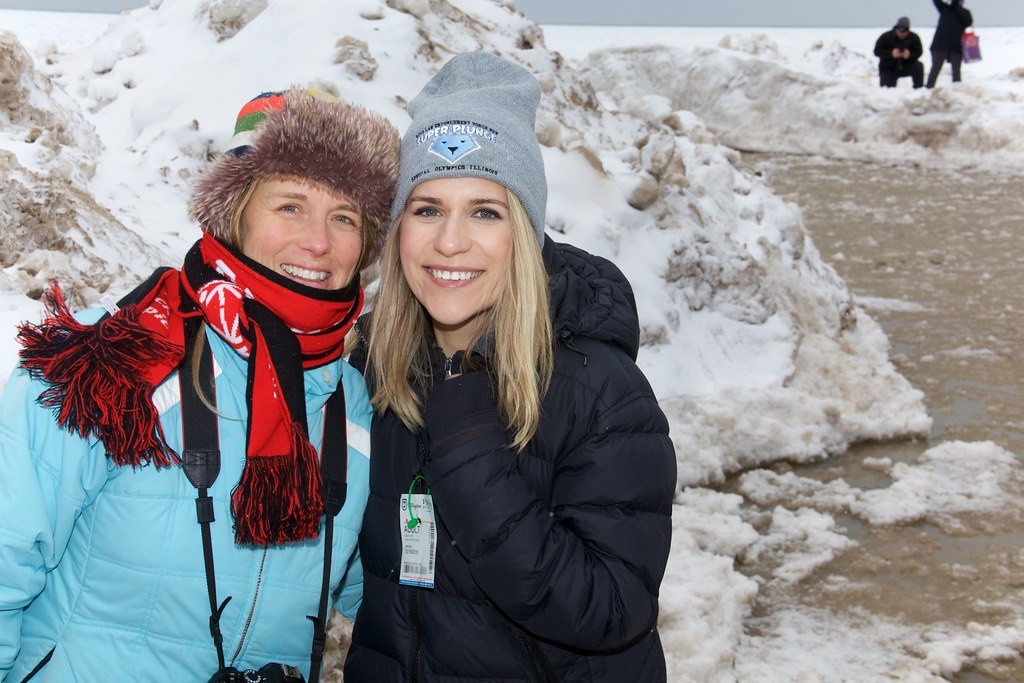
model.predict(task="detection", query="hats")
[192,84,400,272]
[894,16,909,31]
[390,49,549,252]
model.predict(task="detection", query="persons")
[342,48,679,683]
[0,86,402,683]
[874,17,923,88]
[925,0,972,88]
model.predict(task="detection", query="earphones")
[407,517,418,528]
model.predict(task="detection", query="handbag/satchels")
[961,28,982,62]
